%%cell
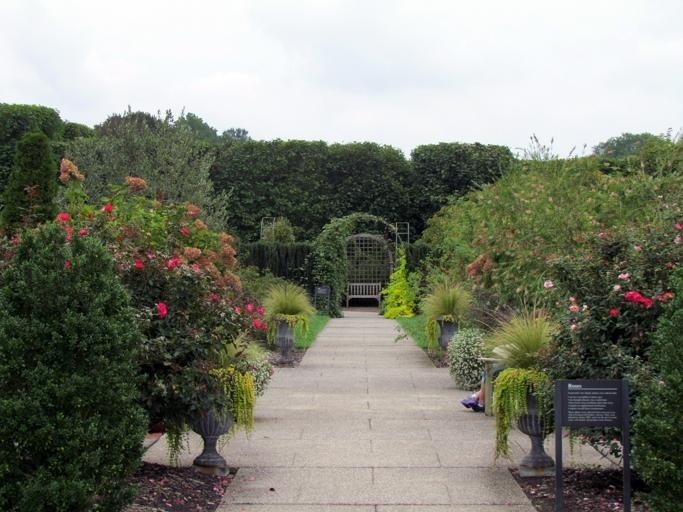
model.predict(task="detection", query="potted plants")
[488,300,563,480]
[424,281,472,355]
[264,283,308,366]
[177,364,243,468]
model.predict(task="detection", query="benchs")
[346,281,382,308]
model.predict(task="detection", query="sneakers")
[461,397,483,411]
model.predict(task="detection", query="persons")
[460,364,500,412]
